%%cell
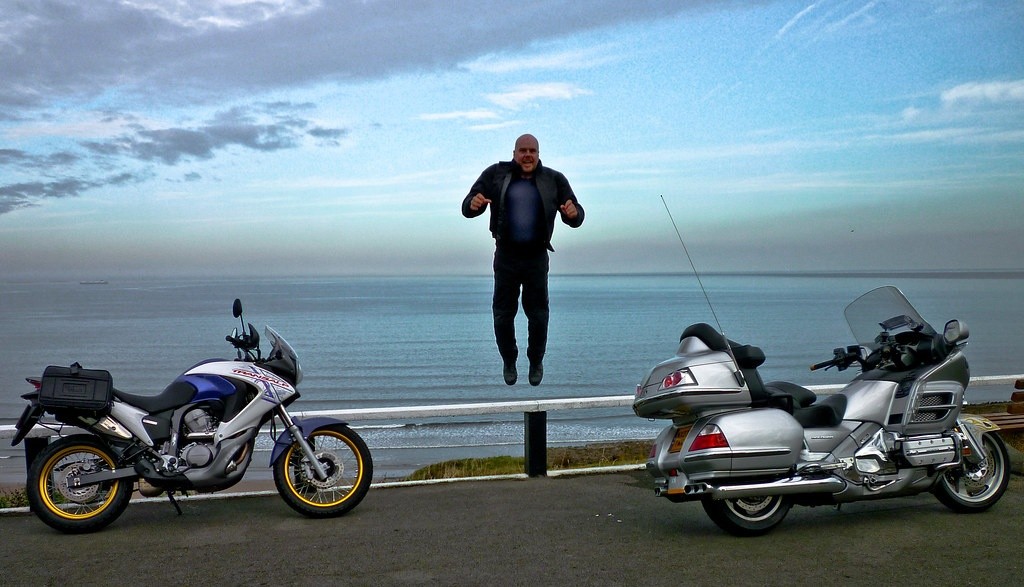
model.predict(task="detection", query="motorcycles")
[10,298,371,535]
[634,193,1013,539]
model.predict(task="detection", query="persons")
[462,134,585,386]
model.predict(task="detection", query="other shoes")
[528,360,543,386]
[504,349,518,385]
